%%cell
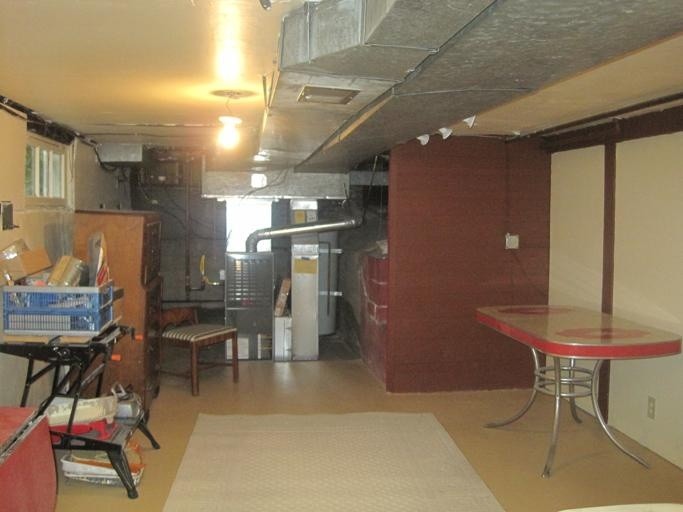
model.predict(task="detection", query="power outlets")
[647,395,656,420]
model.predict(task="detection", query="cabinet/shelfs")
[72,209,165,411]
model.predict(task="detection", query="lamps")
[463,116,477,130]
[439,127,453,140]
[218,94,243,150]
[418,134,430,147]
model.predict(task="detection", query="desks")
[475,303,683,478]
[1,324,160,498]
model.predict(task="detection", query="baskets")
[2,279,114,337]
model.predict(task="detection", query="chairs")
[156,279,239,397]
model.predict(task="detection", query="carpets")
[161,410,505,512]
